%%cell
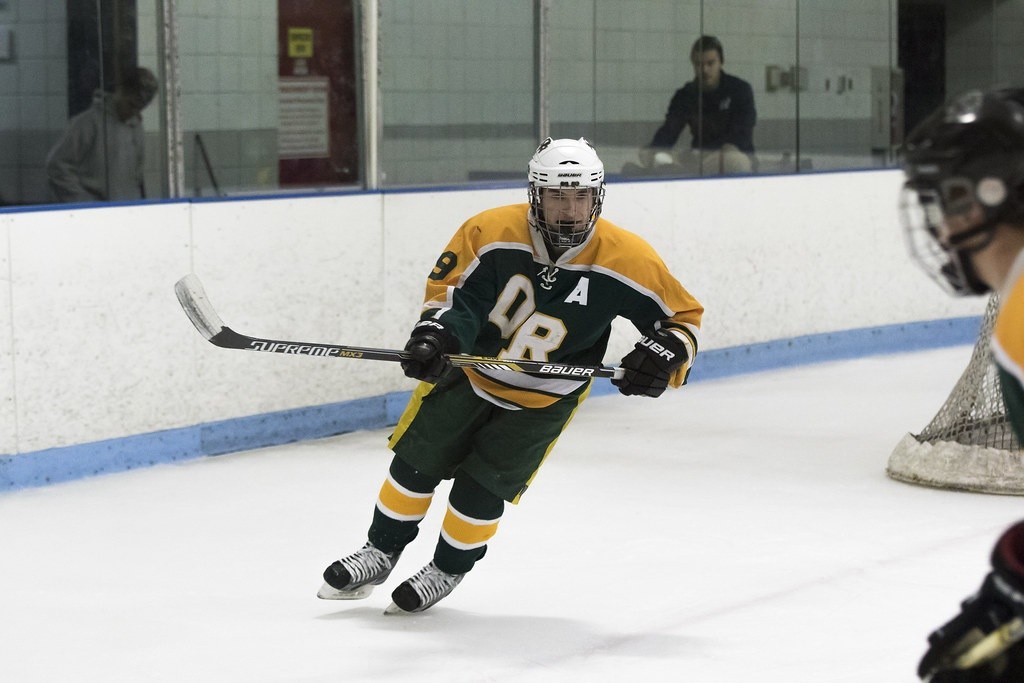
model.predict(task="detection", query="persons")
[895,87,1024,682]
[316,136,703,614]
[47,67,157,203]
[640,35,758,174]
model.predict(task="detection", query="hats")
[108,66,157,103]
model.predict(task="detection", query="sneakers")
[383,544,487,617]
[317,525,419,600]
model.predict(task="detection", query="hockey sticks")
[923,616,1024,683]
[174,273,625,380]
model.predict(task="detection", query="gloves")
[401,318,461,385]
[611,329,690,399]
[918,520,1024,683]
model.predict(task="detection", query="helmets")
[894,84,1024,299]
[527,137,606,247]
